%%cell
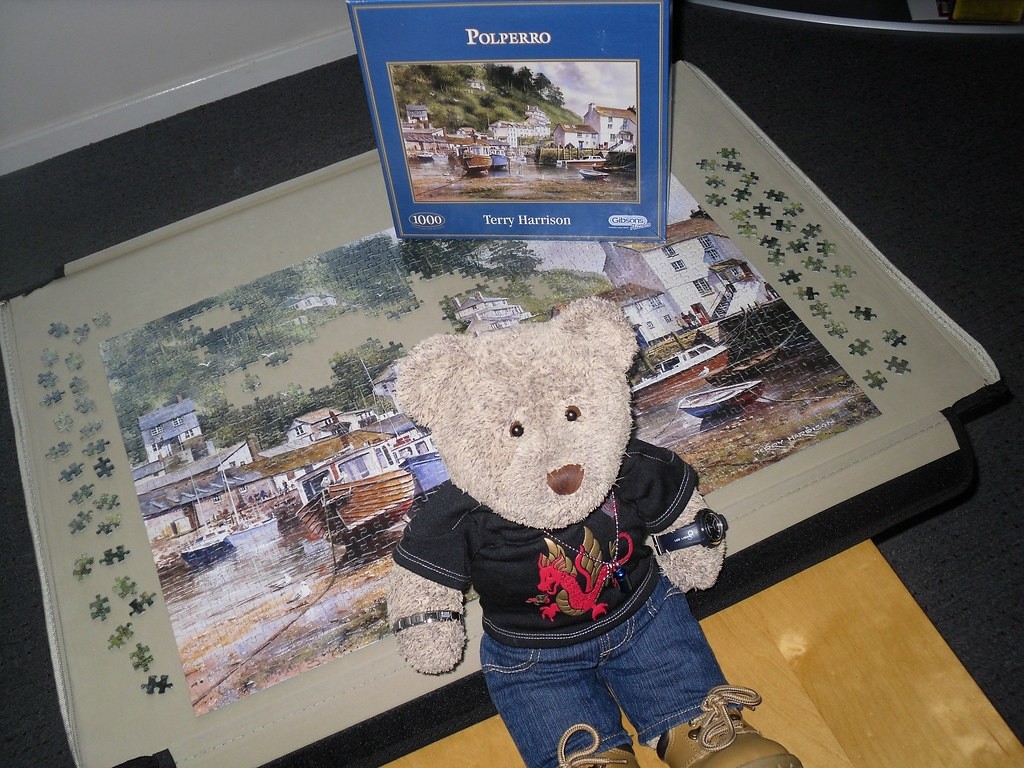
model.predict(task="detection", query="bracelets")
[392,610,465,636]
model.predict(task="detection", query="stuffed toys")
[385,296,802,768]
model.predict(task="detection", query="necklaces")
[541,491,625,586]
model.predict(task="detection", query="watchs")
[652,508,729,556]
